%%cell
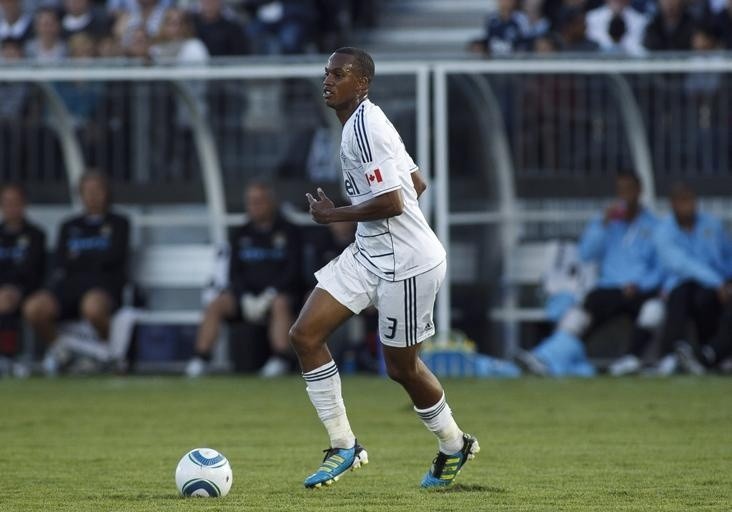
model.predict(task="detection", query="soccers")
[175,448,233,498]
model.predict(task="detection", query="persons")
[183,185,378,381]
[467,0,731,193]
[13,168,129,381]
[287,46,481,490]
[524,175,732,381]
[0,185,50,369]
[0,0,374,202]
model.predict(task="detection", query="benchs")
[130,305,236,371]
[488,303,664,365]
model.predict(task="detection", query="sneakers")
[185,353,214,377]
[260,355,292,379]
[514,351,546,377]
[420,432,481,489]
[303,438,368,489]
[608,343,711,377]
